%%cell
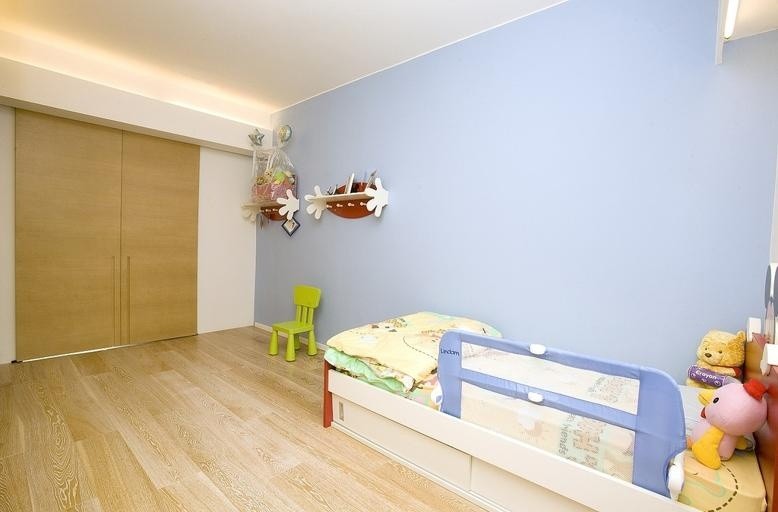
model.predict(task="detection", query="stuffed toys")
[687,330,768,470]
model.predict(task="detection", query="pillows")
[325,311,499,396]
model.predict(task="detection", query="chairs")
[267,285,320,360]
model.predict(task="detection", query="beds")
[322,319,776,505]
